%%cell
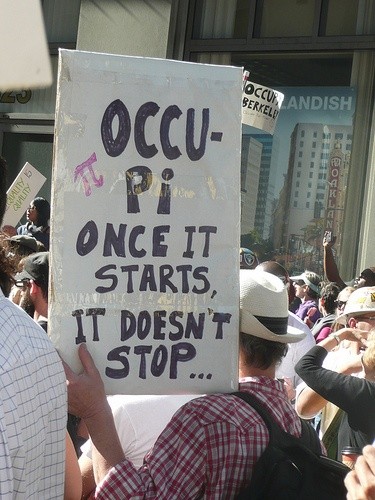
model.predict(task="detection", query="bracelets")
[330,333,342,345]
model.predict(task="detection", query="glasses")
[26,204,36,210]
[358,315,375,324]
[14,279,39,288]
[334,299,348,311]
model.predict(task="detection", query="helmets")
[240,247,260,269]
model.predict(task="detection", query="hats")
[3,234,37,253]
[14,251,49,280]
[240,269,307,343]
[361,267,375,284]
[335,286,375,325]
[289,272,321,295]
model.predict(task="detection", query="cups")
[341,445,362,471]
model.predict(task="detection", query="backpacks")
[229,390,352,500]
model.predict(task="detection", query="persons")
[55,269,326,500]
[0,157,375,500]
